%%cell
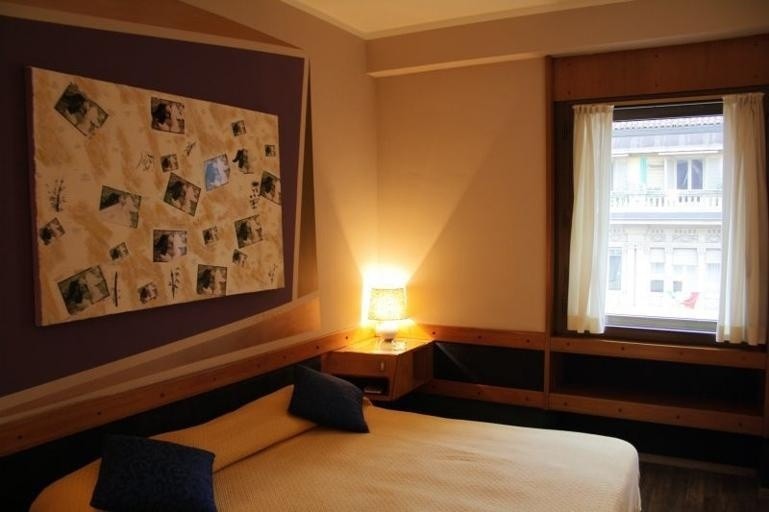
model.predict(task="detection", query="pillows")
[89,433,217,512]
[286,364,369,433]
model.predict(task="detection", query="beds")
[29,383,641,512]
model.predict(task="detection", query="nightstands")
[327,340,434,409]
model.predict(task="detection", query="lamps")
[366,288,409,352]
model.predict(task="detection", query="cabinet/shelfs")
[427,324,769,476]
